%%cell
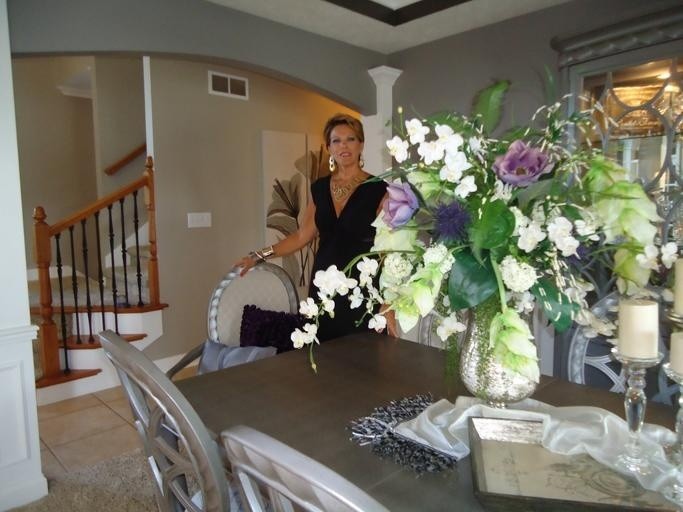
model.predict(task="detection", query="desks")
[157,329,683,510]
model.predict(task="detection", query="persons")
[227,110,401,355]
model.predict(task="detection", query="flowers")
[290,67,678,382]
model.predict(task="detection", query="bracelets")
[253,249,266,262]
[260,245,274,260]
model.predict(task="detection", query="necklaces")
[330,171,368,202]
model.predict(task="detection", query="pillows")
[198,336,266,374]
[240,305,317,353]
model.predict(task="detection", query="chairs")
[96,328,240,510]
[221,423,389,512]
[164,259,682,406]
